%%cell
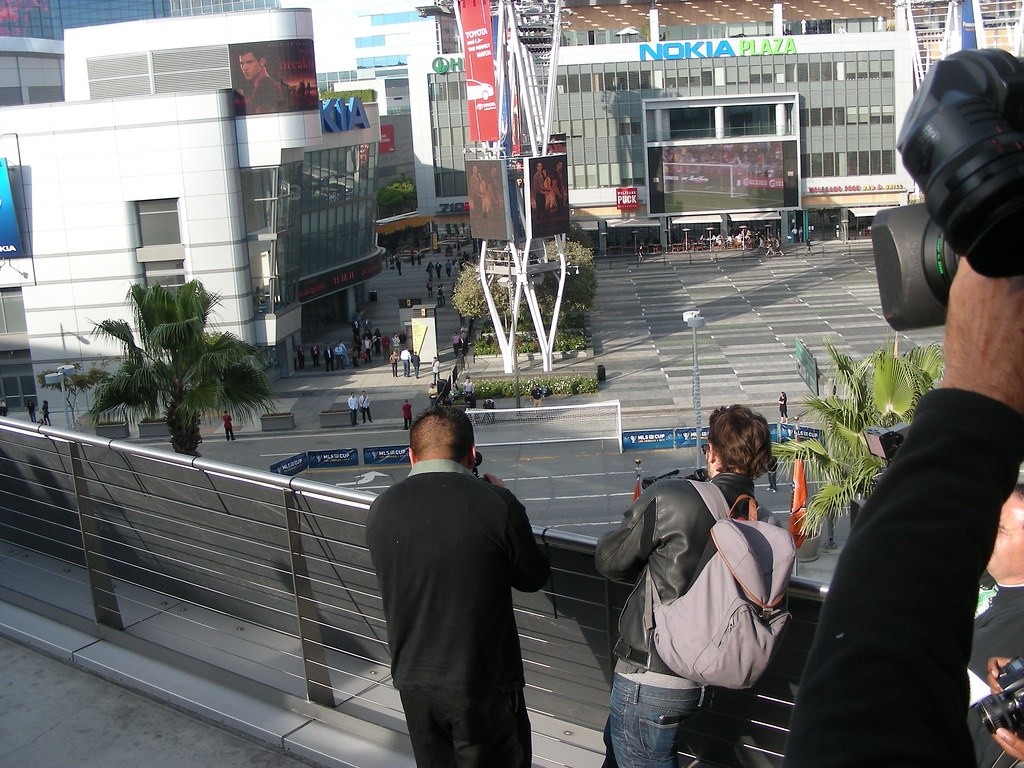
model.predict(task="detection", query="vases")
[260,412,294,430]
[318,410,351,426]
[138,417,171,437]
[797,530,821,562]
[95,420,130,439]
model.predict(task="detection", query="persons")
[348,390,373,426]
[427,251,469,280]
[403,400,413,430]
[410,249,422,266]
[366,406,552,768]
[699,229,785,257]
[452,314,469,358]
[596,404,774,768]
[27,400,36,422]
[429,357,440,409]
[297,315,420,378]
[223,410,235,441]
[531,385,542,417]
[767,456,777,494]
[805,237,814,256]
[0,399,8,417]
[638,245,644,264]
[426,281,445,307]
[779,392,788,423]
[791,226,803,243]
[396,260,402,275]
[42,400,52,426]
[236,44,285,114]
[462,376,495,424]
[781,255,1024,768]
[467,159,568,220]
[665,142,783,166]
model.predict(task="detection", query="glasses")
[701,444,710,455]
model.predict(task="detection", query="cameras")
[869,46,1024,333]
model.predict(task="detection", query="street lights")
[601,232,610,257]
[682,228,689,253]
[632,231,640,256]
[45,365,75,432]
[738,225,748,251]
[664,229,668,259]
[682,309,705,470]
[706,227,714,253]
[764,224,772,240]
[841,219,850,244]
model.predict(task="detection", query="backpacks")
[642,479,797,692]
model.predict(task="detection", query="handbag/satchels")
[359,351,366,359]
[359,407,363,412]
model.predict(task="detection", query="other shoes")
[766,487,774,491]
[772,490,776,494]
[362,421,366,424]
[231,438,235,440]
[227,439,229,441]
[369,420,373,423]
[352,423,359,426]
[402,428,408,430]
[404,375,410,377]
